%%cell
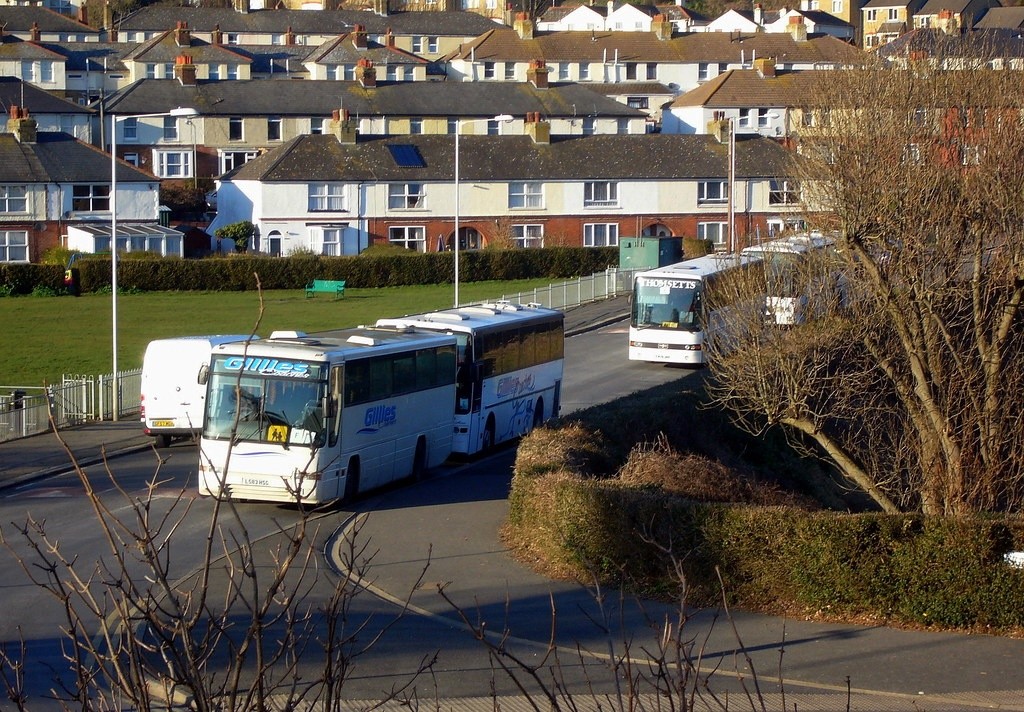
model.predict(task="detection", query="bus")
[628,254,767,364]
[197,325,461,505]
[743,233,850,324]
[374,303,564,455]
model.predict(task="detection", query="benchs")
[305,279,346,301]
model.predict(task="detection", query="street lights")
[454,115,516,300]
[108,107,199,415]
[731,112,781,252]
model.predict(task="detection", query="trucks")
[138,334,260,448]
